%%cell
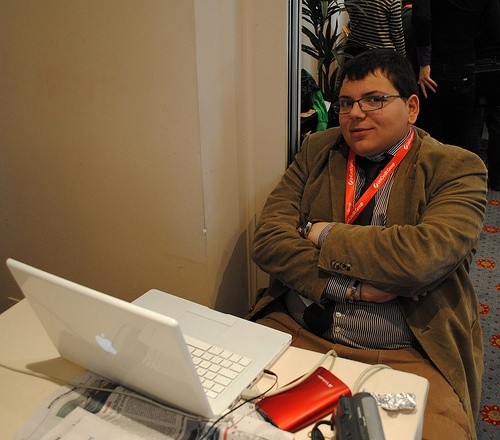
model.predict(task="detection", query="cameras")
[333,392,386,440]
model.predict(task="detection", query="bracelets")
[347,280,360,303]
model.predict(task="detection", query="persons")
[327,0,500,193]
[245,49,487,439]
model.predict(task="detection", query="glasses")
[334,93,404,115]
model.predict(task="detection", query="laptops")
[6,258,293,419]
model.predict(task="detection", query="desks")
[0,298,429,440]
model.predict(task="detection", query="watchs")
[296,218,325,239]
